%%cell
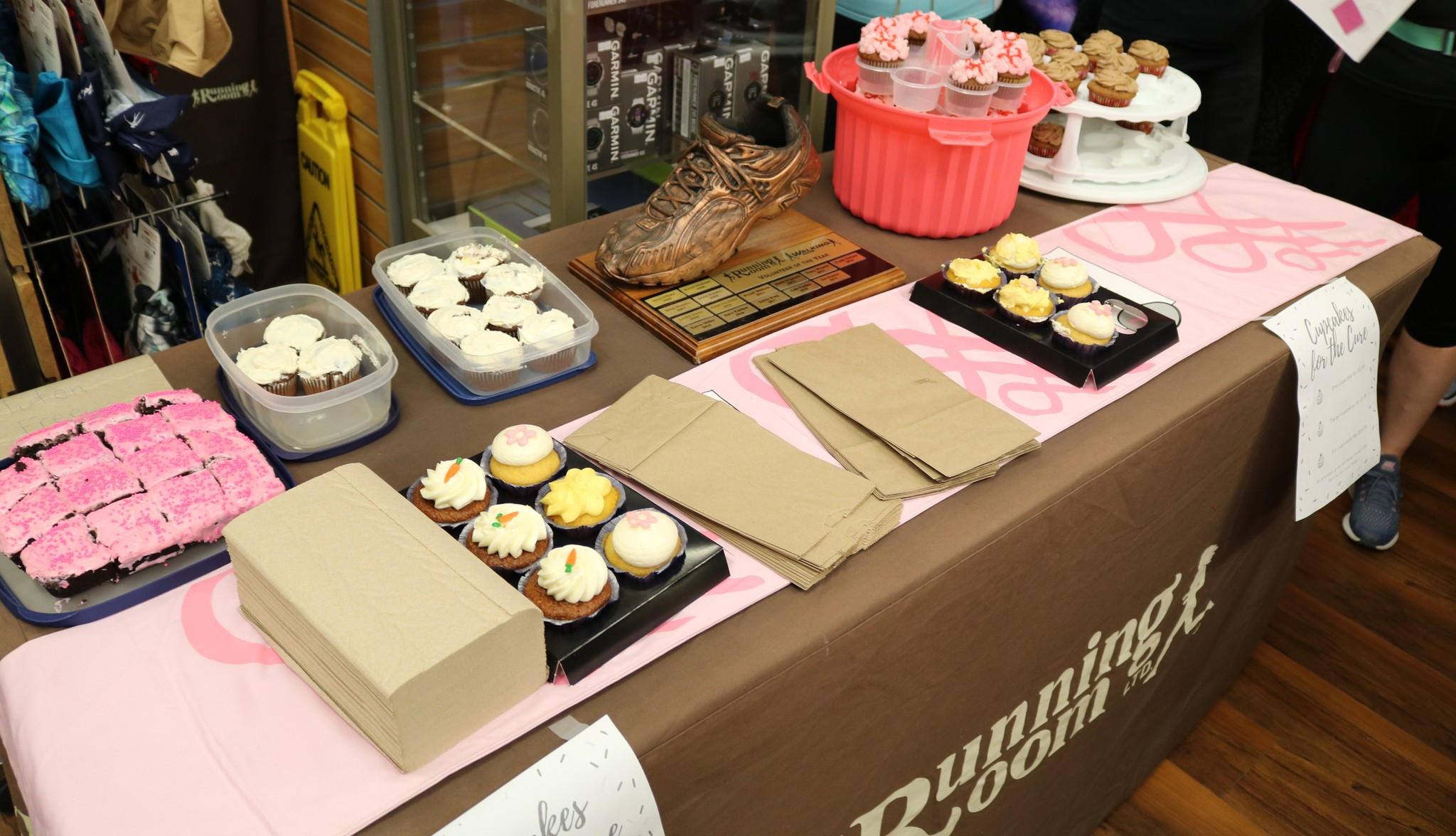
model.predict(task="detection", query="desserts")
[410,423,688,624]
[0,387,285,598]
[946,232,1116,346]
[235,315,360,396]
[855,10,1168,159]
[384,242,574,390]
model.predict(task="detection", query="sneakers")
[595,92,823,286]
[1340,453,1400,550]
[1438,380,1456,407]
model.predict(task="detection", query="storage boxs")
[205,282,400,453]
[523,23,771,176]
[372,226,599,397]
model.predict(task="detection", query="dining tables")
[0,146,1441,836]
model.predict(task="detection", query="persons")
[1268,1,1456,552]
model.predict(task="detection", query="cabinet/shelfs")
[381,0,835,259]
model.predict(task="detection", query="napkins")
[221,462,551,774]
